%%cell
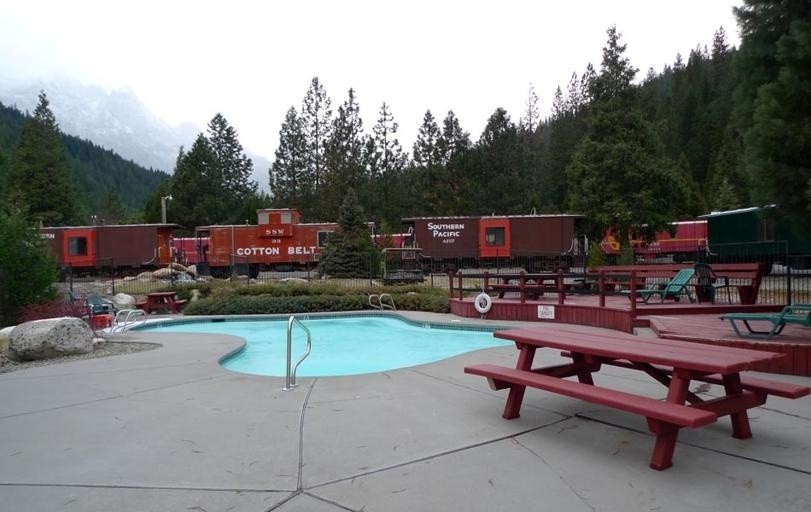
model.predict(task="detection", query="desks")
[145,292,179,312]
[461,321,787,471]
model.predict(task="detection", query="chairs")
[694,263,732,304]
[717,304,811,339]
[85,292,118,323]
[618,267,697,304]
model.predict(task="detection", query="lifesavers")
[475,293,492,314]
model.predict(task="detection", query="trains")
[698,199,811,265]
[24,196,588,274]
[174,220,711,271]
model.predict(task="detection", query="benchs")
[586,260,768,304]
[486,283,546,290]
[462,363,719,429]
[558,347,810,401]
